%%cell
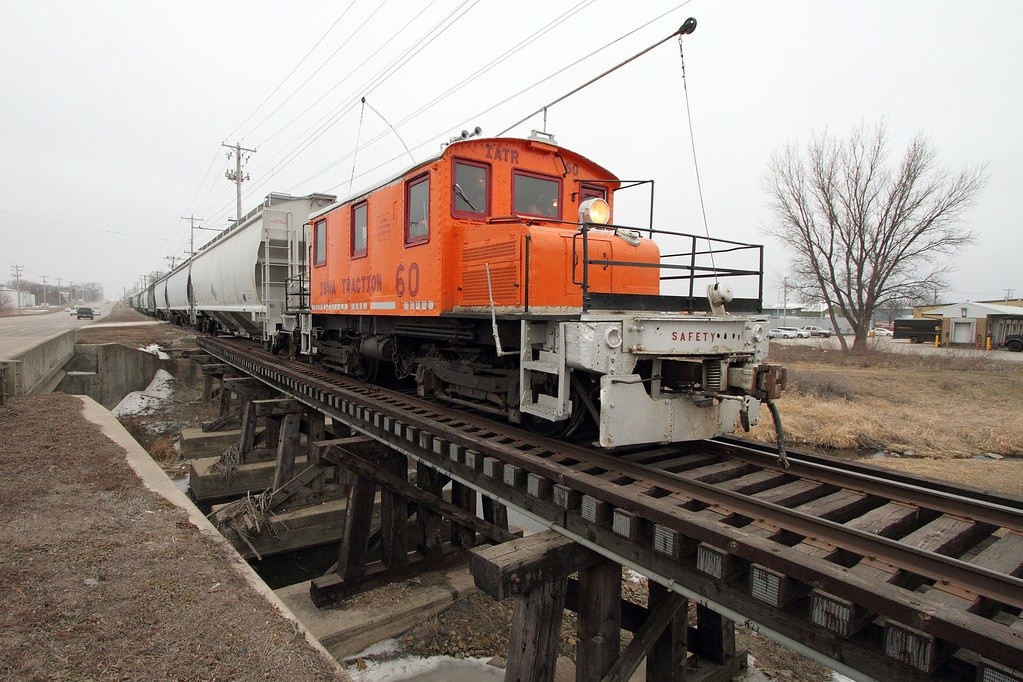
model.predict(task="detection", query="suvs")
[778,327,811,338]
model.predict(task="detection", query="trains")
[128,128,794,470]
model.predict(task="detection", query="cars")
[874,328,894,337]
[70,306,79,316]
[884,326,895,331]
[64,307,71,312]
[77,308,95,319]
[1004,334,1023,352]
[92,308,101,316]
[801,326,832,338]
[769,329,796,339]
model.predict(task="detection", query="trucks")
[893,318,943,344]
